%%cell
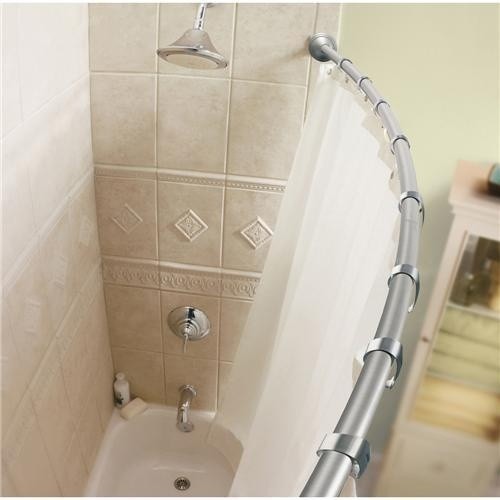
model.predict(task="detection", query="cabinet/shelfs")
[371,215,500,497]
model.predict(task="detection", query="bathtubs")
[84,400,245,497]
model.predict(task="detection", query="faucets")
[174,390,195,434]
